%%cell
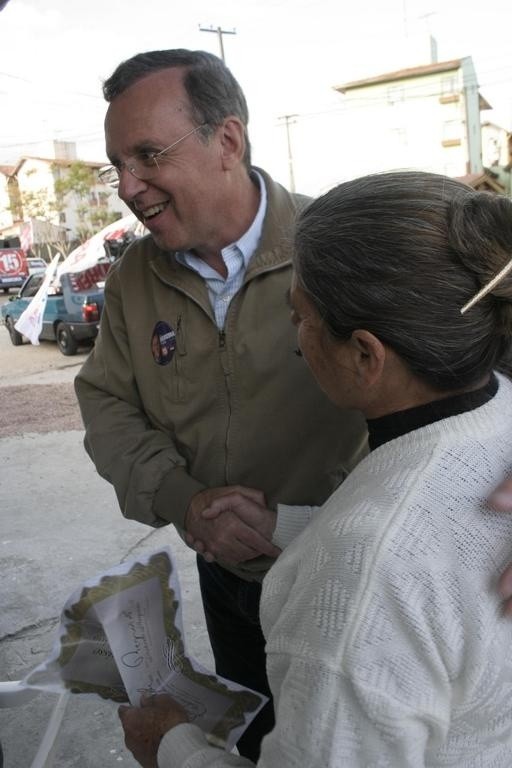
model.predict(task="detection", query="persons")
[73,47,508,767]
[119,170,511,766]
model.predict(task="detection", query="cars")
[1,265,106,357]
[0,256,50,293]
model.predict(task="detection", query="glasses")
[97,121,209,189]
